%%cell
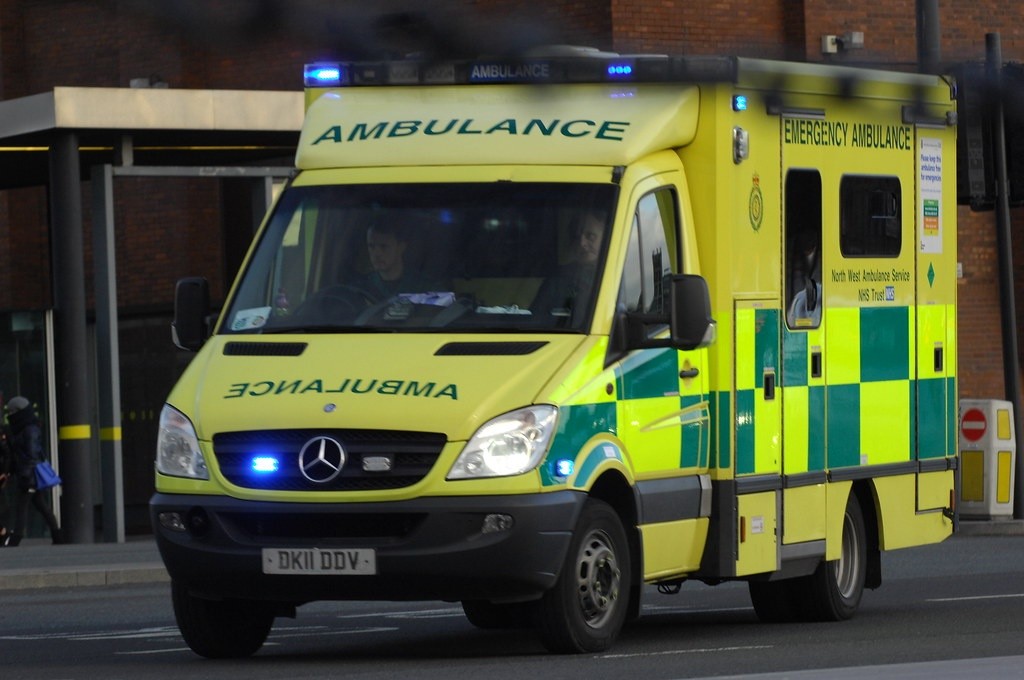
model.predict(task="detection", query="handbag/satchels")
[34,459,61,490]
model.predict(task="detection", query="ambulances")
[148,39,962,659]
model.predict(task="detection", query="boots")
[46,516,63,544]
[8,515,22,546]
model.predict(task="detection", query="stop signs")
[961,407,988,443]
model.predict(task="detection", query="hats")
[7,396,29,415]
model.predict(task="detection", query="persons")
[0,397,67,547]
[528,199,639,315]
[328,217,454,330]
